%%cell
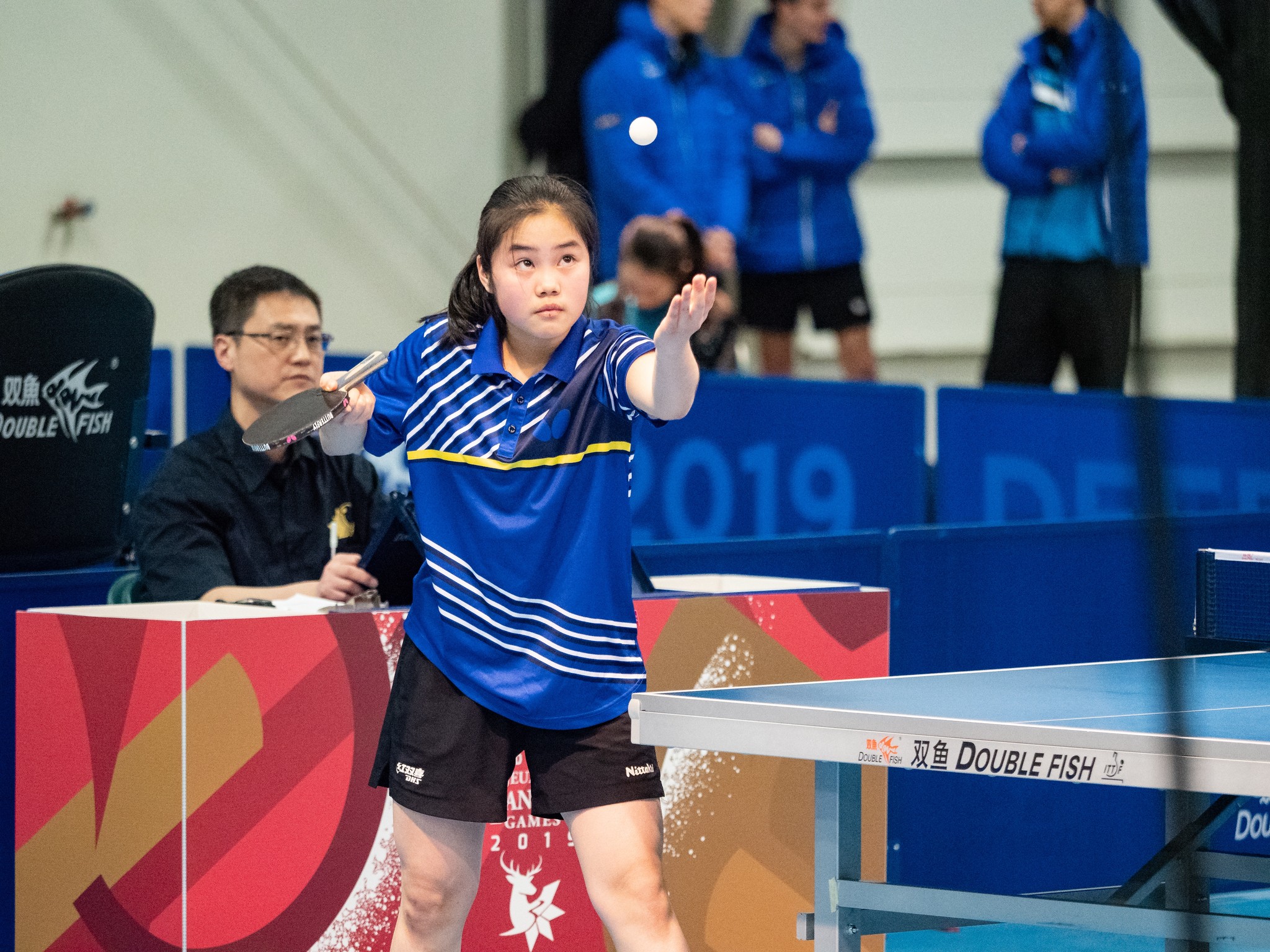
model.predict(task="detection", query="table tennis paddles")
[241,349,389,454]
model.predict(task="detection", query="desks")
[15,576,890,952]
[628,650,1269,952]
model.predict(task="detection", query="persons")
[724,0,879,384]
[973,0,1148,395]
[124,264,381,605]
[316,169,721,952]
[582,0,751,373]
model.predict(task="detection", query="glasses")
[219,325,333,357]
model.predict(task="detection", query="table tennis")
[629,116,659,146]
